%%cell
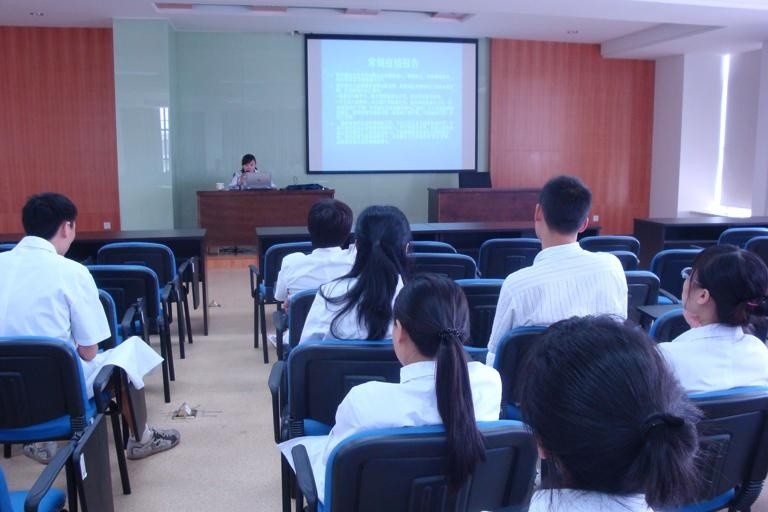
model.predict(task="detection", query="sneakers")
[23,441,59,464]
[266,334,278,348]
[126,426,181,461]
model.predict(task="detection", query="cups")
[216,183,225,190]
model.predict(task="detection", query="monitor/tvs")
[244,172,272,190]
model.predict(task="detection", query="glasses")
[682,268,701,287]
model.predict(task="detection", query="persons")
[230,154,276,189]
[1,192,180,465]
[518,317,699,511]
[486,177,630,355]
[657,248,767,395]
[299,207,416,345]
[273,200,357,313]
[302,272,505,507]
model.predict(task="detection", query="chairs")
[1,244,200,512]
[250,227,767,511]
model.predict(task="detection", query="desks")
[427,189,545,224]
[256,222,603,272]
[634,215,767,270]
[2,229,209,334]
[196,188,334,254]
